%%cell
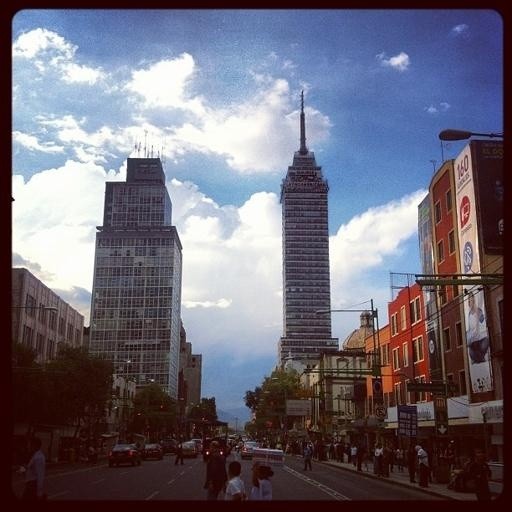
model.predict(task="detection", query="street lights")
[316,309,383,406]
[11,305,58,312]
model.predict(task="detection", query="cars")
[241,445,255,459]
[180,434,240,461]
[142,438,177,460]
[107,444,141,466]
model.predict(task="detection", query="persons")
[174,439,185,464]
[249,462,275,500]
[224,460,248,501]
[303,443,312,471]
[465,452,494,503]
[204,440,227,500]
[52,437,148,467]
[464,290,485,344]
[234,434,328,461]
[17,437,49,500]
[327,437,435,487]
